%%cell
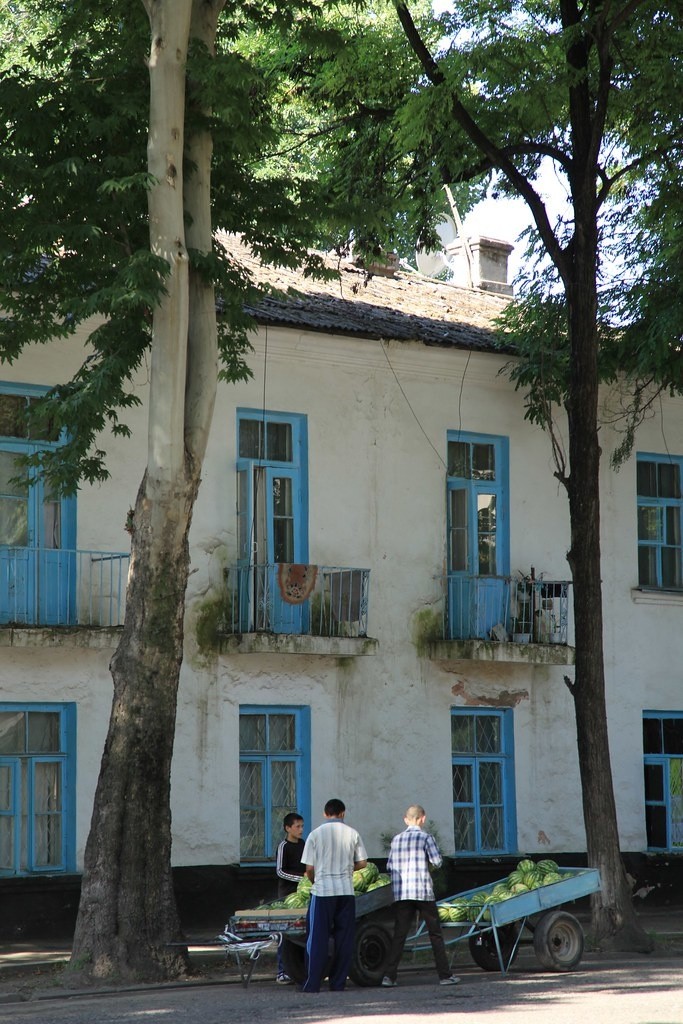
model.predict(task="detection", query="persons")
[276,813,305,984]
[296,799,368,992]
[382,804,461,986]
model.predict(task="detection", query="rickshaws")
[405,867,602,981]
[166,872,395,988]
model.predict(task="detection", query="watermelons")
[437,859,586,922]
[353,862,391,897]
[256,876,312,910]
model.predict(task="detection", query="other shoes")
[276,972,294,984]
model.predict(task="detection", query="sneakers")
[381,974,398,986]
[440,975,461,986]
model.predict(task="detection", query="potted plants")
[516,569,532,600]
[547,615,567,643]
[510,607,532,643]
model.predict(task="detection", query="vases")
[543,600,552,607]
